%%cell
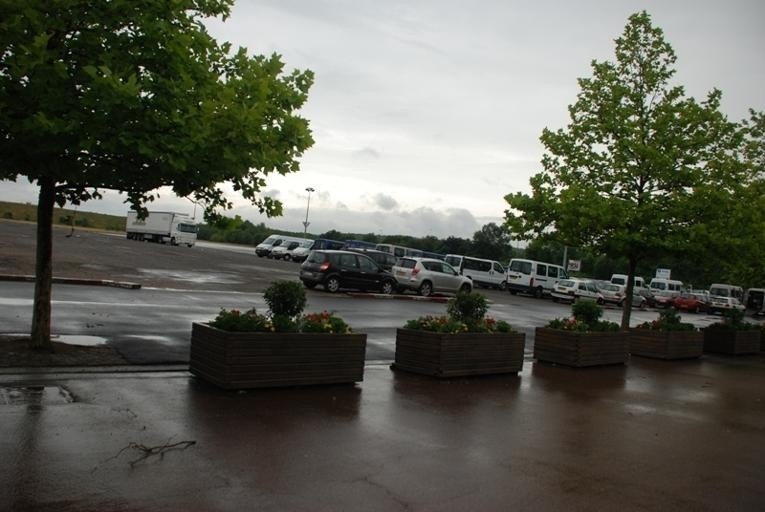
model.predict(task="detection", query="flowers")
[545,298,622,332]
[207,278,356,334]
[706,308,763,330]
[401,290,517,334]
[634,304,698,329]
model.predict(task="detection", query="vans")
[504,257,569,299]
[253,232,446,273]
[442,253,507,291]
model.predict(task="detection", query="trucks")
[124,209,199,249]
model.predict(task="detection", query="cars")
[550,270,764,319]
[391,255,473,300]
[299,248,399,296]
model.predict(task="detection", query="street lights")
[191,198,199,219]
[303,187,315,234]
